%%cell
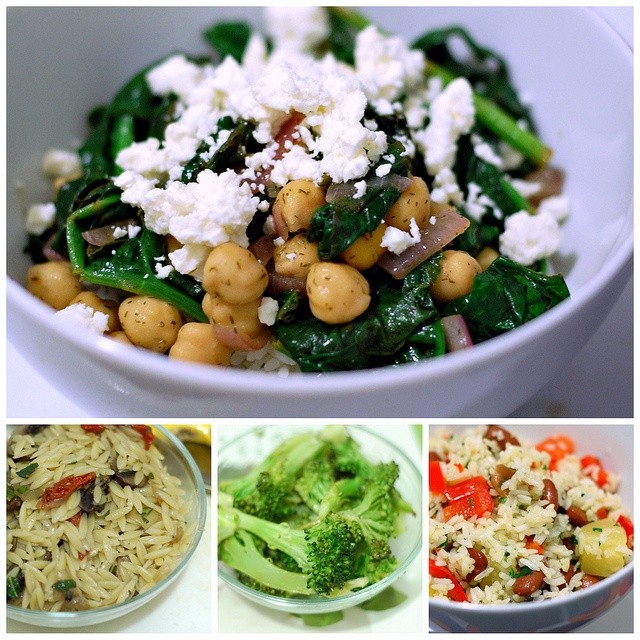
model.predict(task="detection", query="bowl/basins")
[427,424,633,634]
[8,7,635,418]
[212,424,422,615]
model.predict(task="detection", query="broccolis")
[219,427,416,602]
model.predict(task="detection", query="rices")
[430,425,633,602]
